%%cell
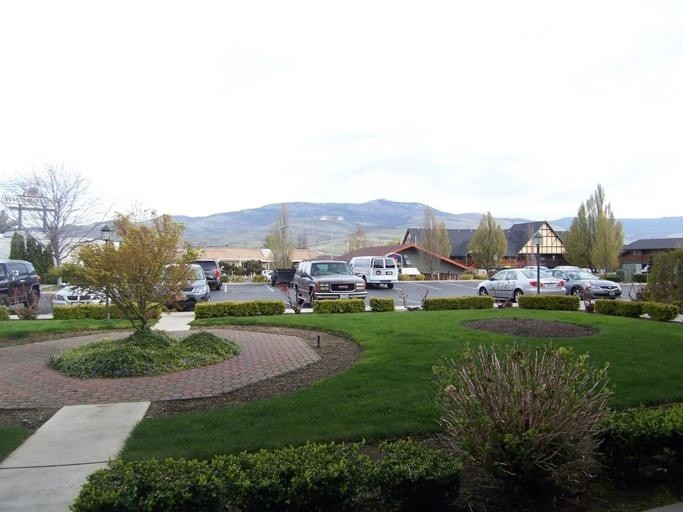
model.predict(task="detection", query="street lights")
[101,225,112,319]
[533,231,543,295]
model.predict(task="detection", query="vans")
[348,256,398,289]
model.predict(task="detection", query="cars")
[476,264,622,304]
[156,264,211,310]
[262,269,275,281]
[51,278,113,309]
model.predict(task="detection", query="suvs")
[187,258,223,291]
[0,260,41,309]
[291,260,368,307]
